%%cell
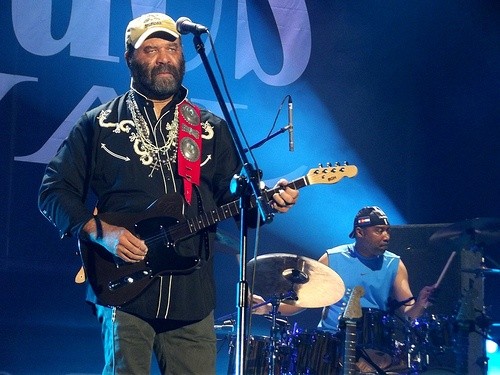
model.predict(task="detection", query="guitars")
[77,160,359,309]
[337,285,366,375]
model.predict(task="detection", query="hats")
[349,206,390,239]
[124,12,182,51]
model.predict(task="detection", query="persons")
[247,205,433,329]
[38,13,298,375]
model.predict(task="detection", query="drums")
[407,312,454,356]
[278,330,342,375]
[337,307,396,375]
[226,334,286,375]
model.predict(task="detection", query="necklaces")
[126,89,182,162]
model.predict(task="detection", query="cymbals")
[426,216,500,252]
[458,267,500,274]
[245,252,346,309]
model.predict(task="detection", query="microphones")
[386,296,413,312]
[288,96,294,152]
[176,17,209,34]
